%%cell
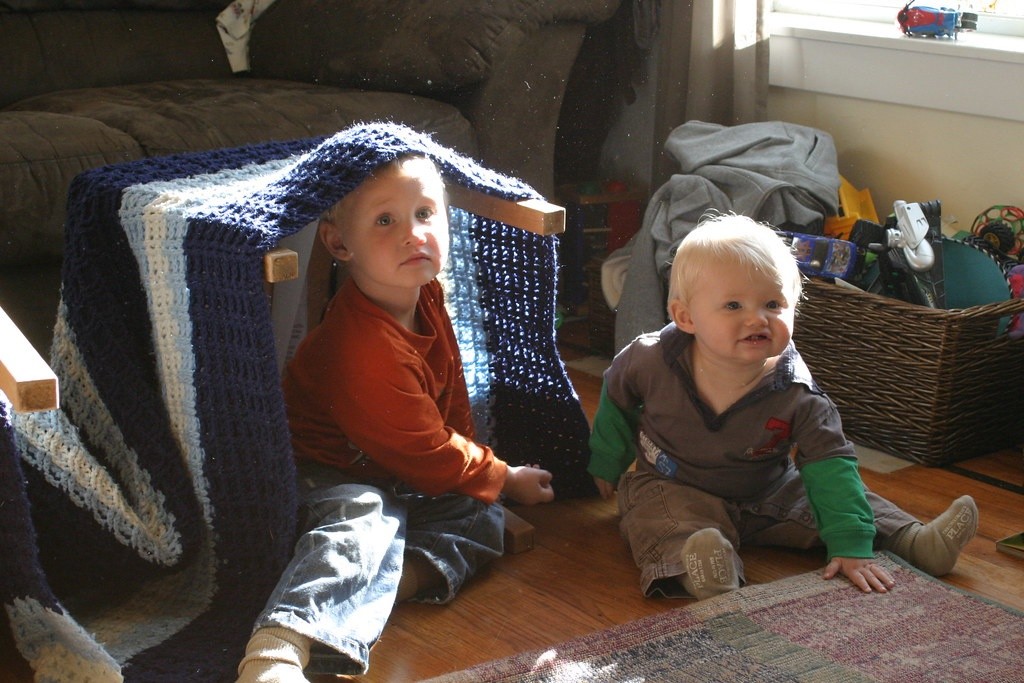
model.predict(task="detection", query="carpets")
[417,551,1024,683]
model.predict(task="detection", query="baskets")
[791,277,1024,467]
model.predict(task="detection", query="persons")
[588,212,980,602]
[235,149,556,683]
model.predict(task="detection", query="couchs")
[0,0,619,364]
